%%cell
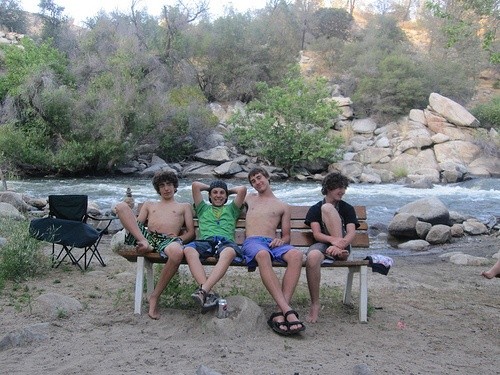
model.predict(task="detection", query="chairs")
[29,195,120,272]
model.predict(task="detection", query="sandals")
[267,312,290,335]
[283,309,306,334]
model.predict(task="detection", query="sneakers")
[191,284,207,304]
[201,294,218,314]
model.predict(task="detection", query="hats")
[209,182,228,204]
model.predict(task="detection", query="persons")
[116,171,196,320]
[241,168,306,336]
[184,180,247,311]
[304,172,360,322]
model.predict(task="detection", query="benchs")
[119,202,371,324]
